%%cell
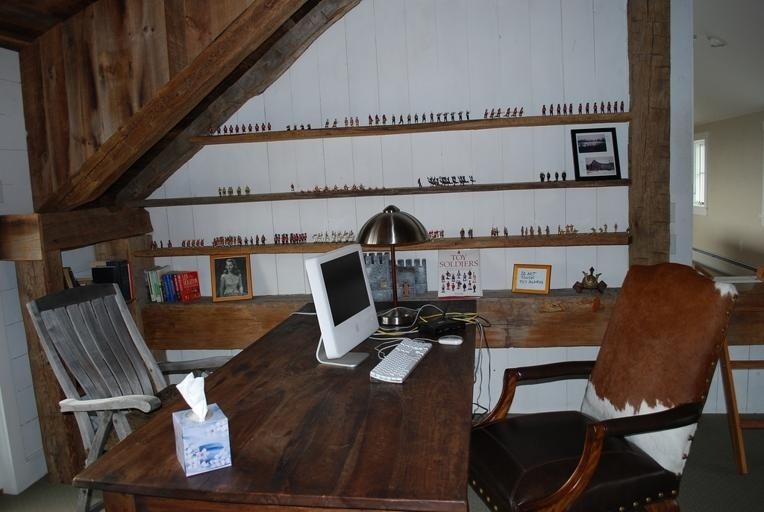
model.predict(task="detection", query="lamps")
[356,206,430,327]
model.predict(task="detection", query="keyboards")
[369,337,432,384]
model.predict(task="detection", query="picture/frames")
[570,127,621,181]
[210,254,254,302]
[511,264,553,295]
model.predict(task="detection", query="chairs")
[463,260,740,511]
[26,283,242,512]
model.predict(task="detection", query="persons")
[219,258,244,297]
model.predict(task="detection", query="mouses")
[437,333,464,347]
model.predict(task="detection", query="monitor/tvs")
[304,243,380,368]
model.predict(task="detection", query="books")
[144,265,201,304]
[92,259,136,305]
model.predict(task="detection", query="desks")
[71,304,478,511]
